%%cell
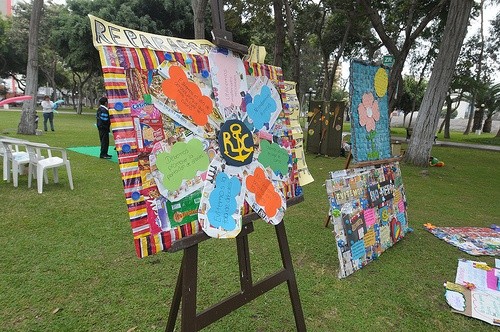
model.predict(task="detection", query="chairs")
[0,135,74,193]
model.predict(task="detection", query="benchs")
[406,127,438,143]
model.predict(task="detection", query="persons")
[41,95,55,132]
[35,111,40,129]
[96,97,112,159]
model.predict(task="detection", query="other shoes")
[100,154,111,159]
